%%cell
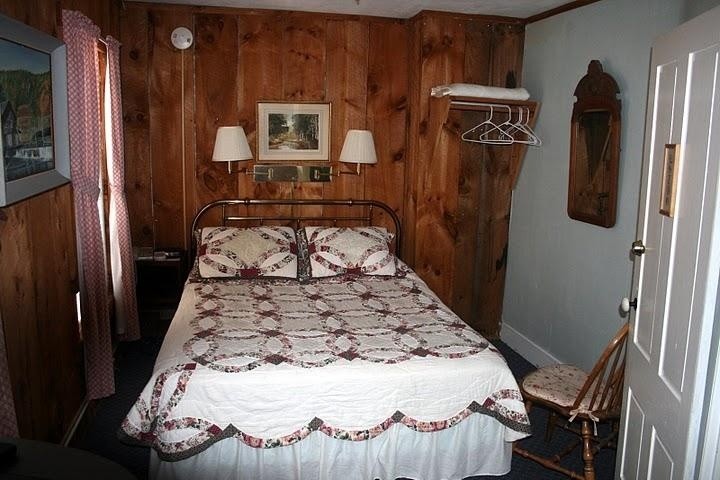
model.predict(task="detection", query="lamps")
[315,127,383,181]
[210,123,274,183]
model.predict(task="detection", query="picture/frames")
[0,12,75,209]
[656,143,682,221]
[254,98,333,162]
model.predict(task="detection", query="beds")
[115,196,535,480]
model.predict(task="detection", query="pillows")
[187,222,300,283]
[294,226,400,281]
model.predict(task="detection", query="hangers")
[461,102,544,151]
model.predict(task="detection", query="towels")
[432,82,529,101]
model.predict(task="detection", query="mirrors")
[565,56,625,229]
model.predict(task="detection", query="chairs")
[512,324,634,480]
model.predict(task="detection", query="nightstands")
[134,249,186,328]
